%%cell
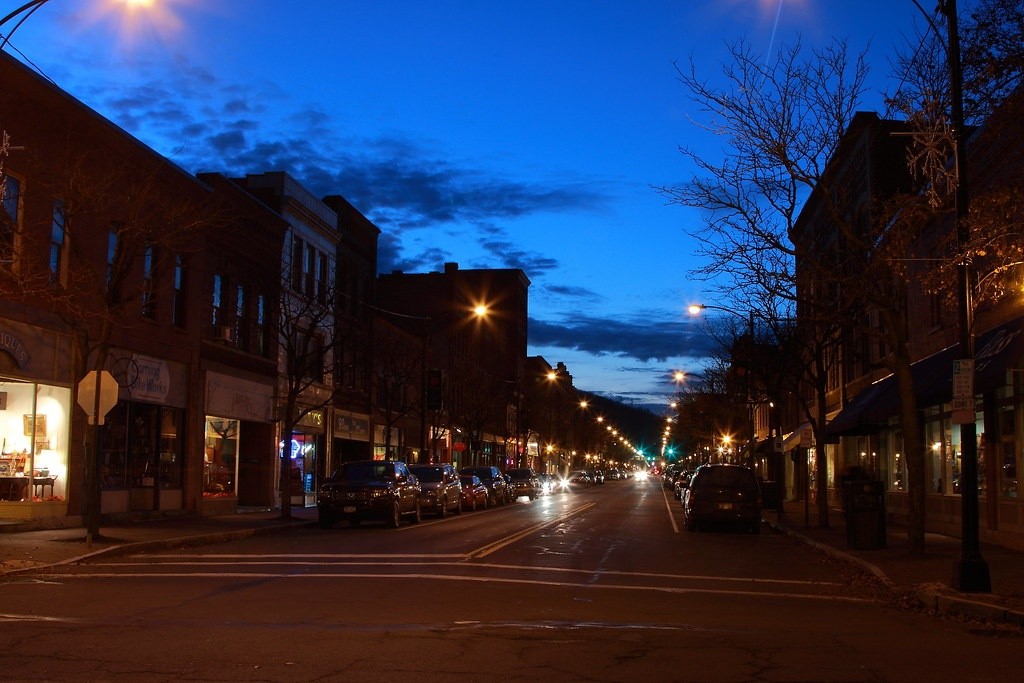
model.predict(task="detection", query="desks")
[26,475,58,498]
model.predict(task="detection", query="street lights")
[420,296,493,466]
[515,371,635,469]
[673,372,713,462]
[684,301,756,475]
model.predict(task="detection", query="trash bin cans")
[844,480,887,550]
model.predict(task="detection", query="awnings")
[825,316,1024,437]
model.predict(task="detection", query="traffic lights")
[636,449,643,455]
[668,446,674,455]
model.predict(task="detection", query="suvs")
[505,467,540,500]
[462,466,509,506]
[317,458,422,529]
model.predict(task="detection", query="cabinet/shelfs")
[207,416,237,439]
[0,476,29,501]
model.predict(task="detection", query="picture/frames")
[24,414,46,437]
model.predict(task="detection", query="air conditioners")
[213,327,230,340]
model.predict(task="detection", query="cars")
[537,466,635,489]
[460,475,490,511]
[680,464,765,534]
[407,465,465,516]
[650,463,694,505]
[502,473,517,502]
